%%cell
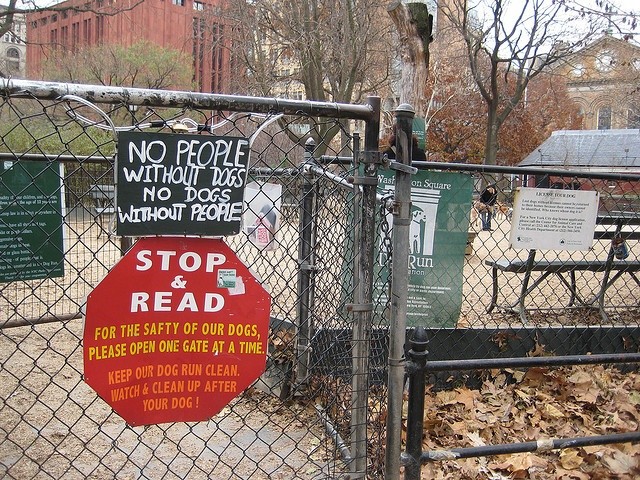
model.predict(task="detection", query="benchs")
[485,216,640,325]
[89,184,114,239]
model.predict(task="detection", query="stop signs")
[84,237,270,427]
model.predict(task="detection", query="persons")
[479,183,498,232]
